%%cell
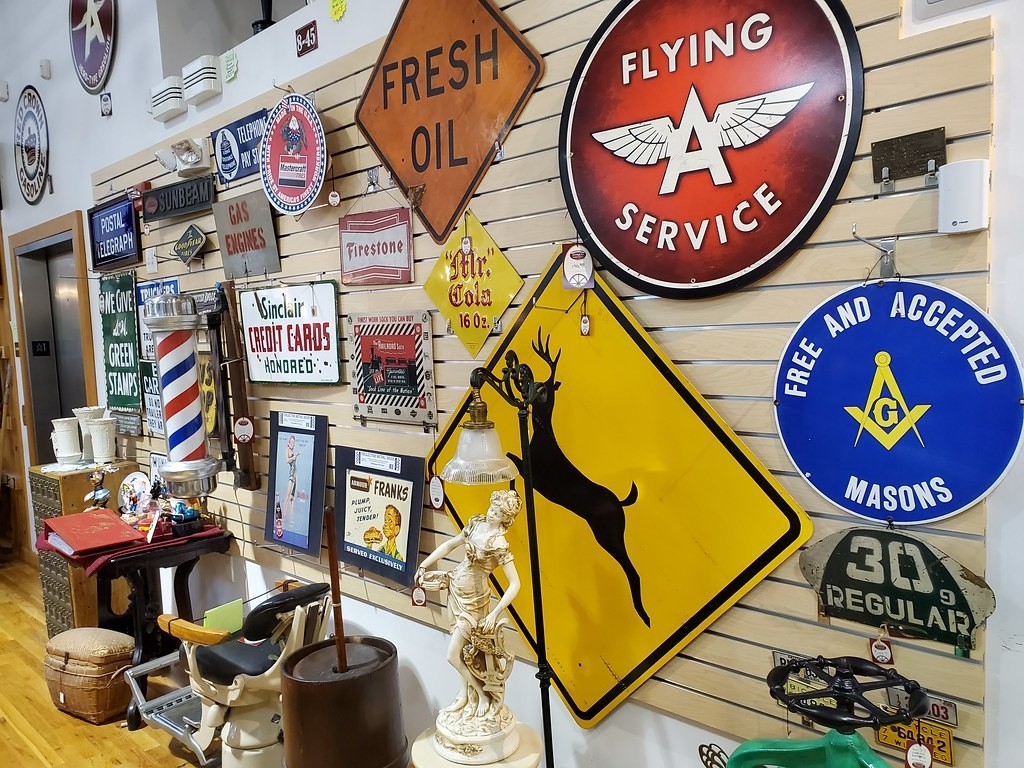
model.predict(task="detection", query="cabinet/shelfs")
[29,460,140,638]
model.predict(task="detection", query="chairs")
[124,580,330,768]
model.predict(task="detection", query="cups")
[86,418,117,465]
[72,406,107,460]
[50,417,81,464]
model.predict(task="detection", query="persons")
[416,490,523,717]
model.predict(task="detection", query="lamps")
[440,351,555,768]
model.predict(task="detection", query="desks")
[87,514,232,731]
